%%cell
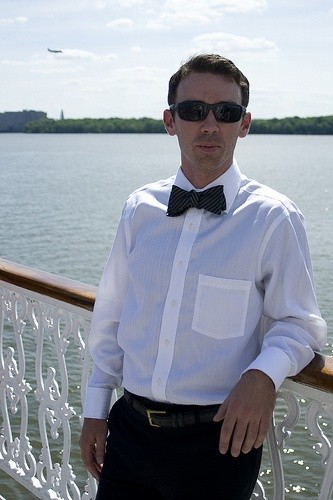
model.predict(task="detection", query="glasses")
[167,98,249,124]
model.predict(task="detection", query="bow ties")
[165,182,227,218]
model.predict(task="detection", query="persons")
[79,53,327,500]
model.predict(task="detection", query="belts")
[121,386,224,431]
